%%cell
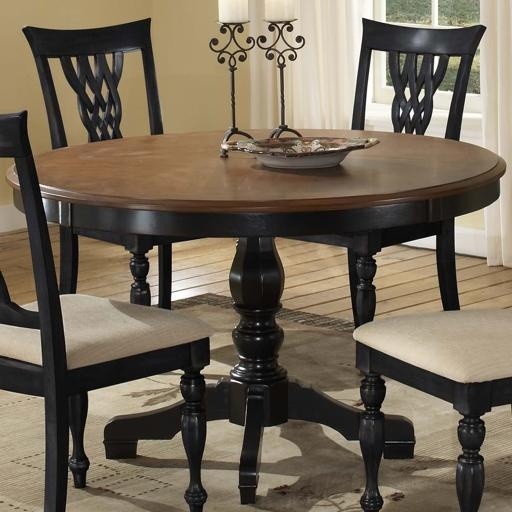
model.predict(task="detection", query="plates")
[220,134,382,172]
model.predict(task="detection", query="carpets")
[0,293,512,512]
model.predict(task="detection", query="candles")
[265,0,300,21]
[218,0,248,23]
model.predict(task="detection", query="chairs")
[352,308,512,512]
[22,17,207,310]
[0,110,214,512]
[275,17,487,329]
